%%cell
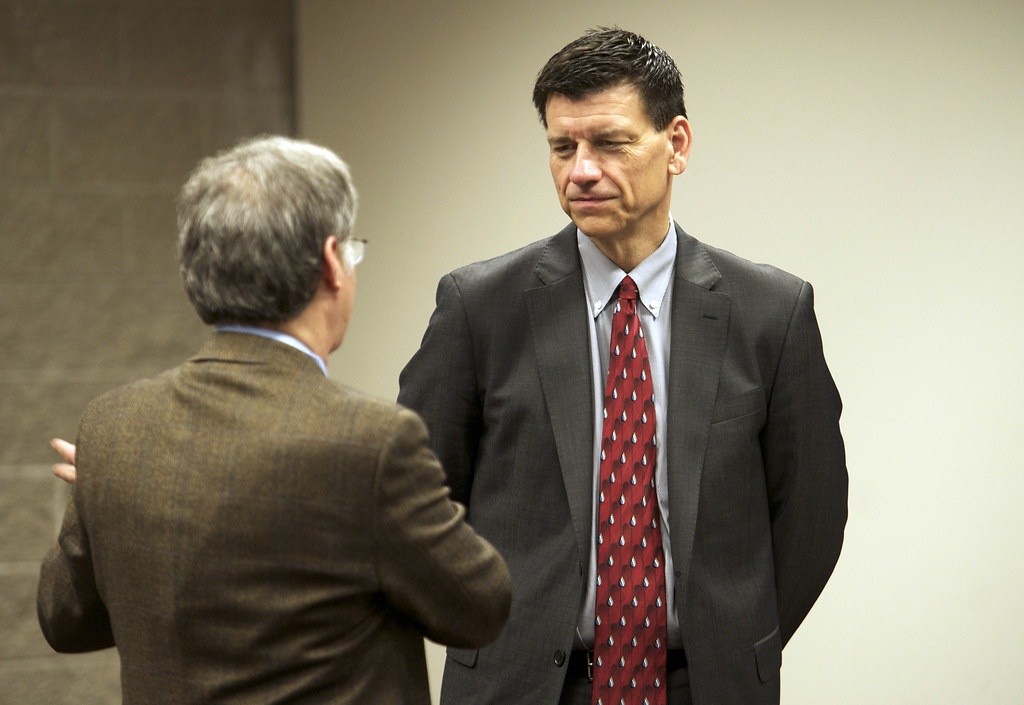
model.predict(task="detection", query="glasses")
[332,235,367,268]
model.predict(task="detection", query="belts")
[566,648,688,682]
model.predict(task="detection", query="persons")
[37,135,512,705]
[396,26,850,705]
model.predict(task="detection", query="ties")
[591,276,668,705]
